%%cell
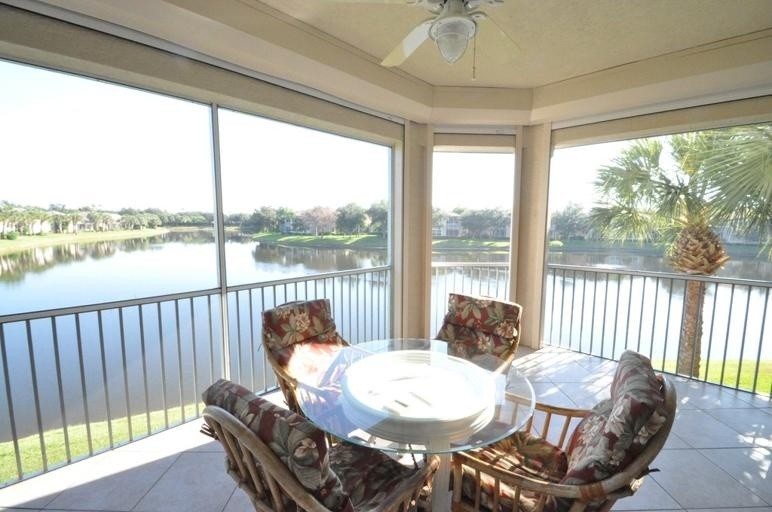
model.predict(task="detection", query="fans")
[380,0,523,75]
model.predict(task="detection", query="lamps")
[427,0,478,65]
